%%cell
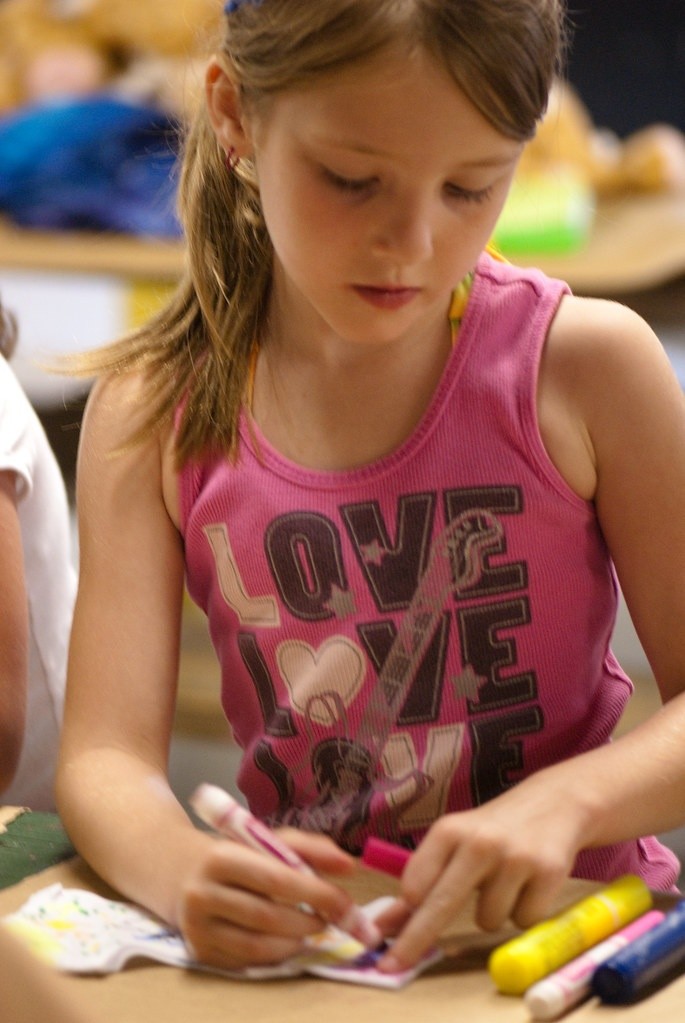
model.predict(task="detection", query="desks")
[0,806,685,1023]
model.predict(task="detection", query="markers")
[486,871,685,1022]
[188,781,391,958]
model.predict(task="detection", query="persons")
[50,0,685,973]
[0,351,78,814]
